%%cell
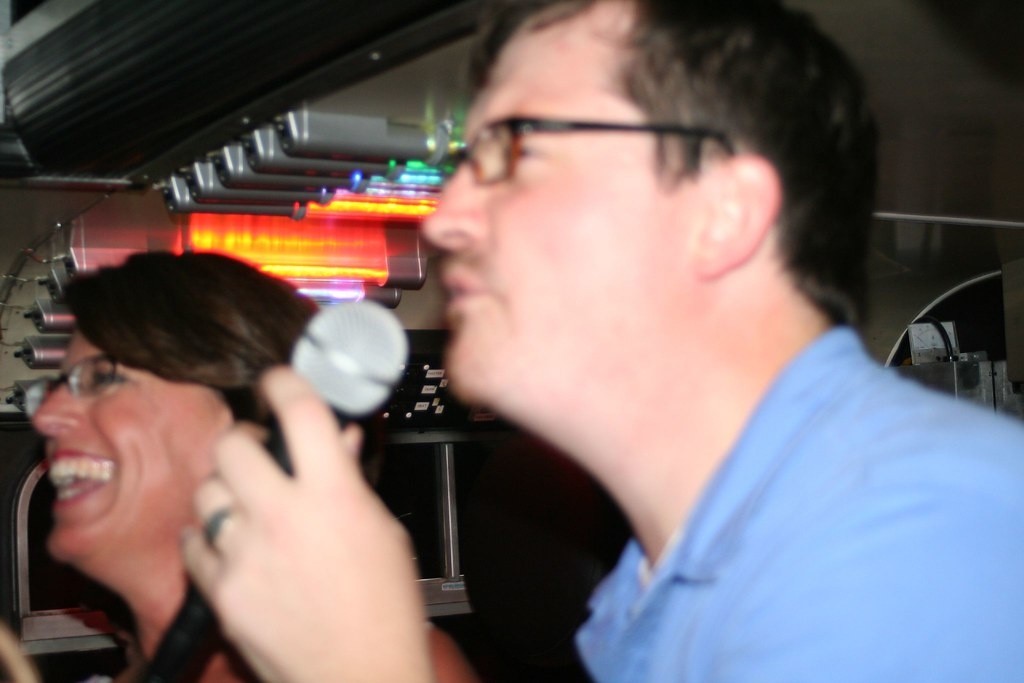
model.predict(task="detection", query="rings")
[202,501,243,549]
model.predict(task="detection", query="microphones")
[136,299,412,683]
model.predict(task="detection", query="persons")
[181,0,1024,682]
[2,247,480,681]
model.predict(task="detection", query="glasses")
[22,350,190,420]
[441,117,735,186]
